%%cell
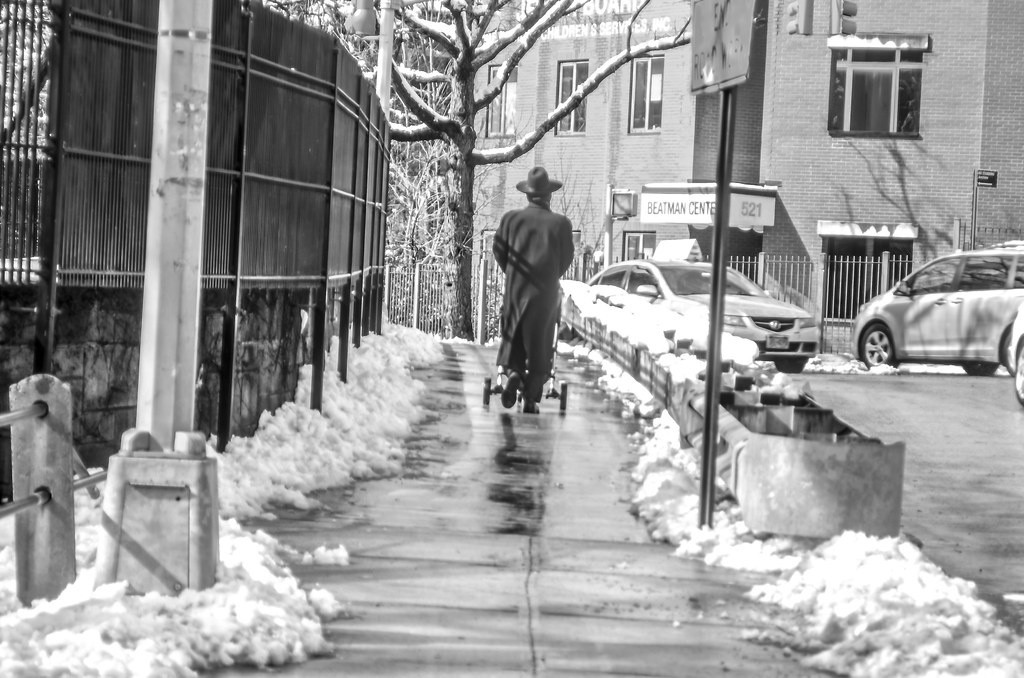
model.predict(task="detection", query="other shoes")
[501,370,520,408]
[524,402,539,413]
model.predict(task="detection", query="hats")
[515,167,563,194]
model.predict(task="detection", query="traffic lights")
[830,0,858,37]
[786,1,814,35]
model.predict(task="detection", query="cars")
[850,246,1024,377]
[1008,299,1024,405]
[582,259,821,375]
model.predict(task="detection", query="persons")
[492,167,575,414]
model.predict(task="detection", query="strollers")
[482,293,568,410]
